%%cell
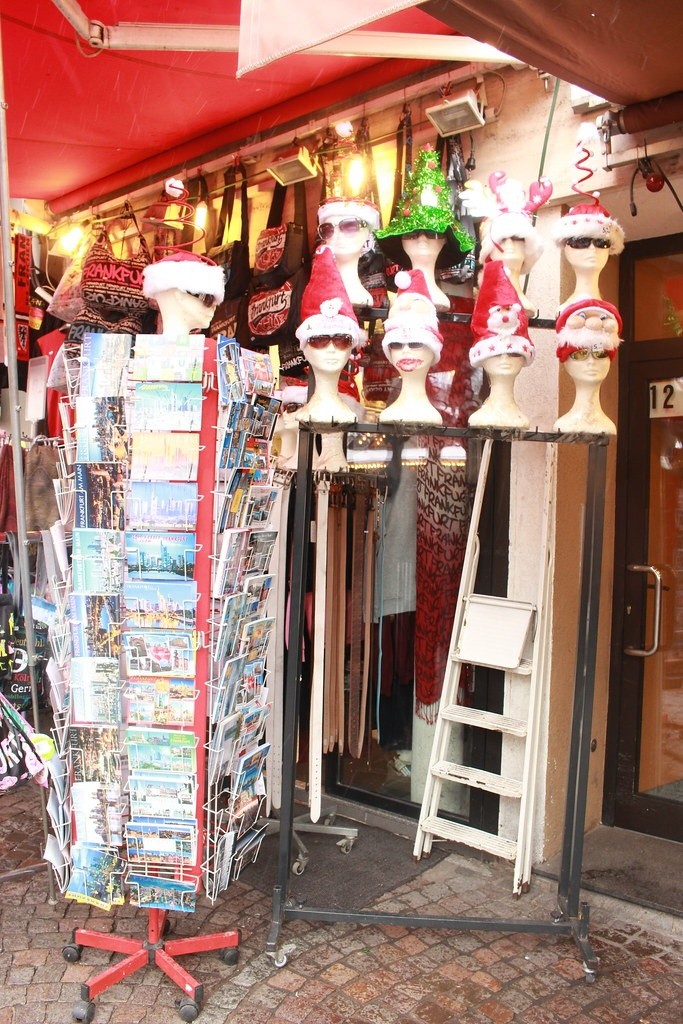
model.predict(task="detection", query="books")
[32,332,281,913]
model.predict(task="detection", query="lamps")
[265,146,319,186]
[138,190,188,230]
[424,82,486,138]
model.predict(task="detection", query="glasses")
[569,350,608,360]
[504,238,524,243]
[310,335,353,350]
[189,291,217,309]
[388,342,423,349]
[568,238,612,250]
[403,230,447,240]
[317,220,372,241]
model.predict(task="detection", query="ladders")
[411,440,559,898]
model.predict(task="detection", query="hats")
[374,142,475,281]
[381,270,442,365]
[296,244,359,350]
[470,260,536,369]
[555,346,618,361]
[553,299,624,335]
[458,173,553,273]
[318,170,381,230]
[553,202,626,255]
[142,251,225,307]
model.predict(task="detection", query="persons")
[557,300,618,436]
[143,256,224,336]
[554,126,625,318]
[316,195,380,305]
[470,259,536,428]
[275,247,366,471]
[379,269,445,423]
[458,172,552,318]
[376,142,475,312]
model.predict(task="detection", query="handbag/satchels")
[46,231,84,322]
[78,203,150,314]
[199,163,307,348]
